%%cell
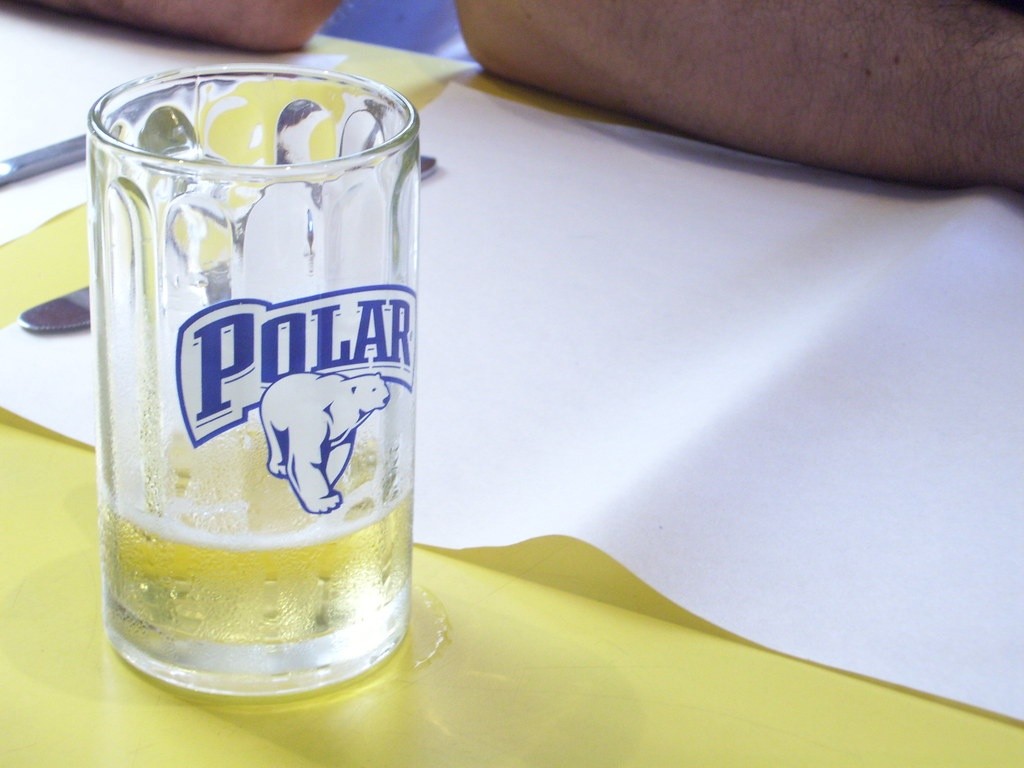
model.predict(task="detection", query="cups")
[87,66,420,697]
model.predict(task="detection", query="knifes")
[17,154,438,332]
[0,51,352,186]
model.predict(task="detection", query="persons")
[0,0,1024,189]
